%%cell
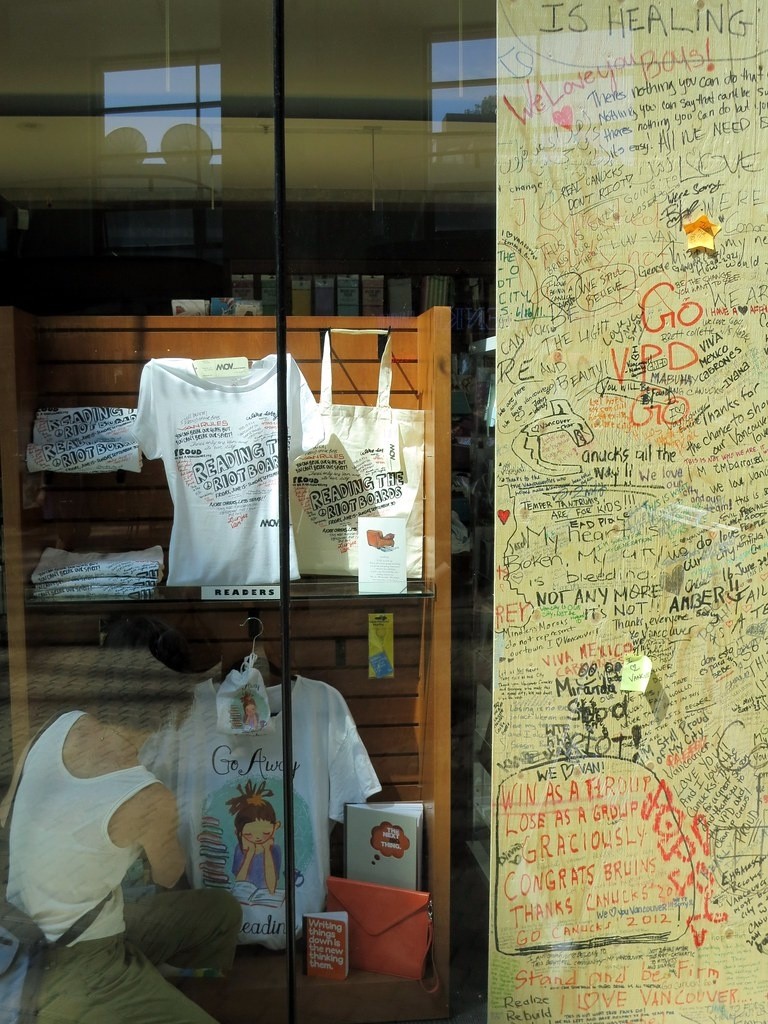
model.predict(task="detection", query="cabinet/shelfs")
[0,307,453,1024]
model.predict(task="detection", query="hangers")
[221,617,296,684]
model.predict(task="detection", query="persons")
[1,616,243,1024]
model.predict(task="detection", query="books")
[302,800,429,979]
[167,267,488,359]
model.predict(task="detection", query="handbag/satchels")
[1,893,50,1024]
[287,329,426,580]
[326,875,441,993]
